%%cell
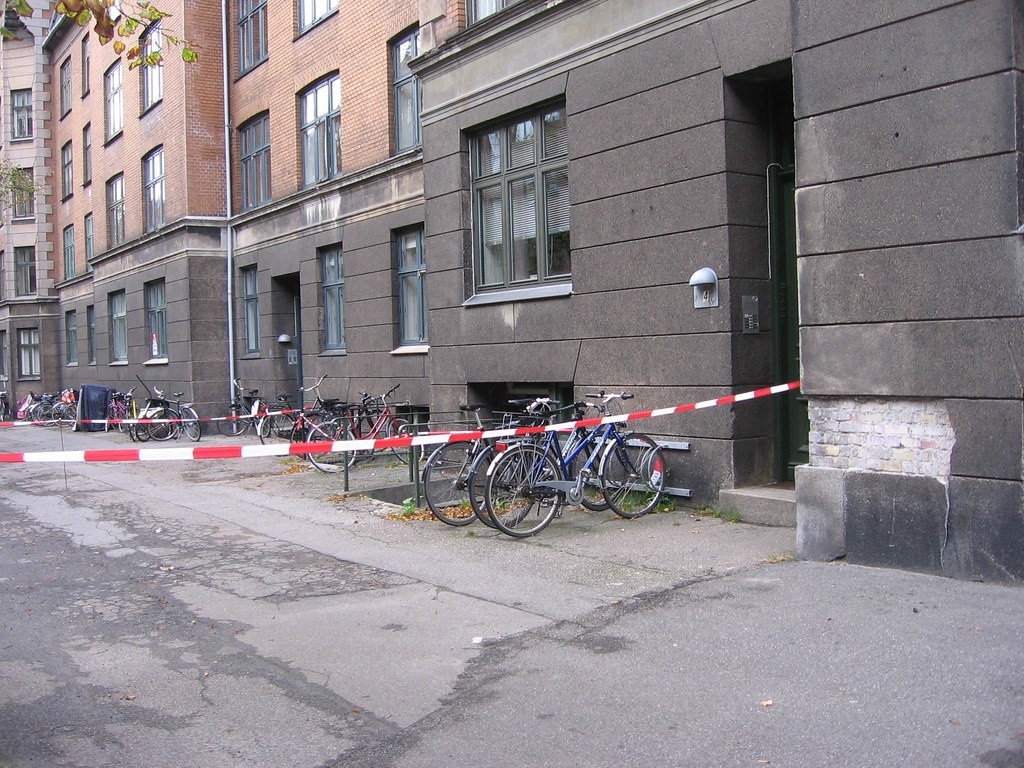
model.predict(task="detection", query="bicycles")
[23,386,202,443]
[217,377,271,438]
[257,374,425,473]
[420,389,669,538]
